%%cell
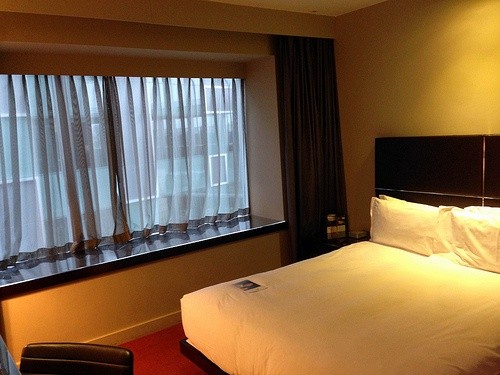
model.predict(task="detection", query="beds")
[180,187,500,375]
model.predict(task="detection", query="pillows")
[368,195,500,273]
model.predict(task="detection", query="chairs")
[19,343,134,375]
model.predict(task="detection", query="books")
[227,279,269,293]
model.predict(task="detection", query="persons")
[235,282,253,290]
